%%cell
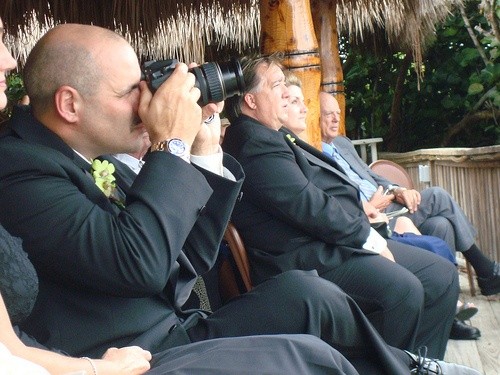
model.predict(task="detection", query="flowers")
[92,160,115,198]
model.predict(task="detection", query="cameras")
[141,58,246,106]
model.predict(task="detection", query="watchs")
[151,137,190,161]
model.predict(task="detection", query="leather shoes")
[404,345,481,374]
[448,318,481,340]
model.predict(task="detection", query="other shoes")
[456,302,477,321]
[476,262,500,296]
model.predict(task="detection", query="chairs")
[369,160,476,296]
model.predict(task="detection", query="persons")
[0,13,500,375]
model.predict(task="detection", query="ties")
[334,151,377,201]
